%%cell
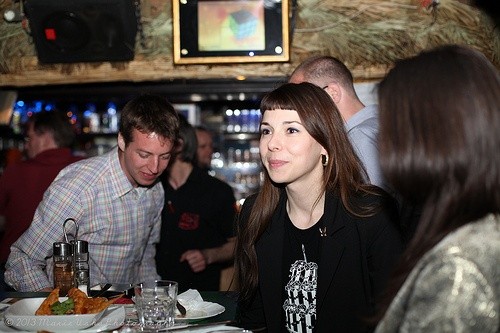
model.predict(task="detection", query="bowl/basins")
[4,296,108,331]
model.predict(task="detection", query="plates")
[154,298,225,324]
[0,303,125,333]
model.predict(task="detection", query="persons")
[290,55,386,190]
[155,122,239,291]
[224,82,408,333]
[0,111,88,292]
[193,125,212,173]
[5,98,180,291]
[372,44,500,333]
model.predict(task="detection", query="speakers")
[21,0,137,64]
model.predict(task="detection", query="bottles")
[69,239,91,297]
[52,241,75,297]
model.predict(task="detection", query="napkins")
[176,288,204,315]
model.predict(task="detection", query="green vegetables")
[49,297,74,315]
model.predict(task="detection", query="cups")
[133,279,178,333]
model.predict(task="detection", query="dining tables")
[0,291,253,333]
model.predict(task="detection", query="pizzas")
[34,285,124,316]
